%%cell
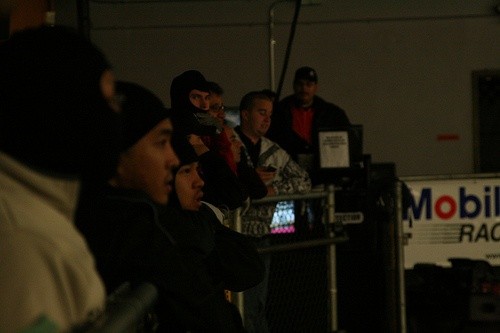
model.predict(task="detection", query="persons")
[232,92,312,333]
[267,66,352,187]
[0,24,155,333]
[77,69,278,333]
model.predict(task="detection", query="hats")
[102,79,169,169]
[294,66,318,83]
